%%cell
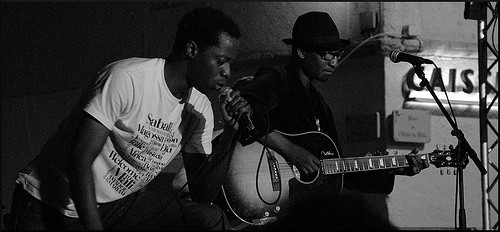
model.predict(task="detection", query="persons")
[218,11,432,232]
[9,7,240,231]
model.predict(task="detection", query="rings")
[417,168,420,173]
[245,100,247,105]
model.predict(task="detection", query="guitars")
[220,130,469,225]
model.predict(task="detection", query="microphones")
[390,50,433,64]
[220,86,255,131]
